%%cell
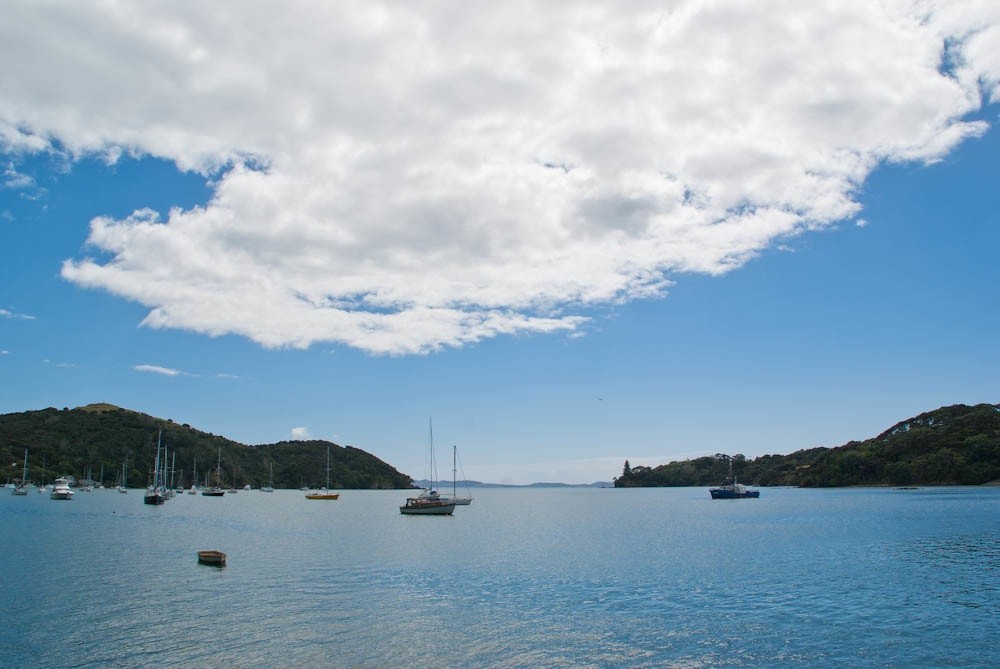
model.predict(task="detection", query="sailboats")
[11,447,30,496]
[144,431,274,505]
[80,464,129,492]
[419,416,472,505]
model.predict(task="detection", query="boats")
[399,497,455,516]
[710,457,759,499]
[51,479,75,500]
[307,494,339,502]
[198,550,225,563]
